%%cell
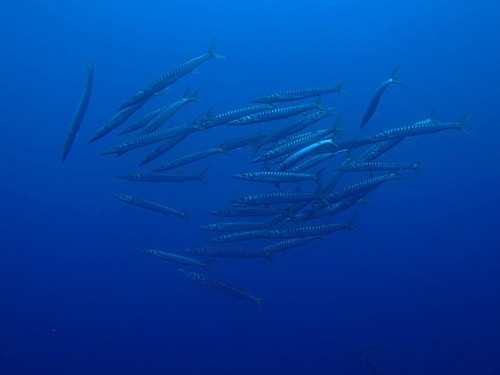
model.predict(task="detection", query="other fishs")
[59,39,470,305]
